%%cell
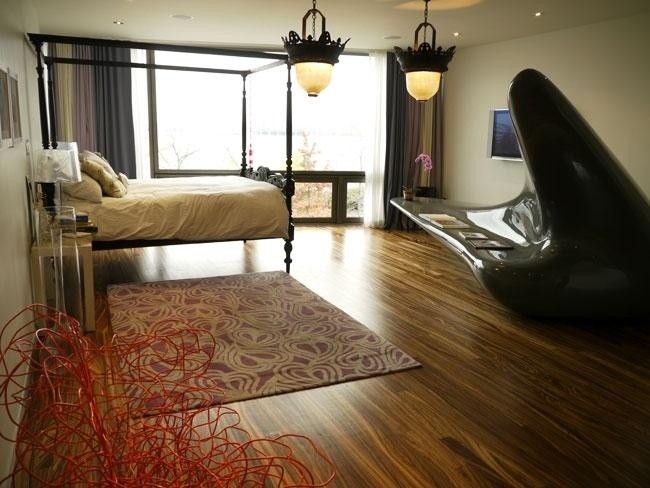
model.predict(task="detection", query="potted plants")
[403,185,415,201]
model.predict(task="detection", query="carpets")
[105,270,423,420]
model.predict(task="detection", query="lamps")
[30,141,80,216]
[391,0,457,104]
[281,0,350,98]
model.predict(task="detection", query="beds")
[45,167,296,273]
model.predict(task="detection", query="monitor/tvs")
[486,107,524,162]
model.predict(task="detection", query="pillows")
[57,147,127,206]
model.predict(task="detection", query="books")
[54,211,97,239]
[418,213,514,250]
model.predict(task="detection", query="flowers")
[413,152,434,186]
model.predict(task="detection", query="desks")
[29,233,96,333]
